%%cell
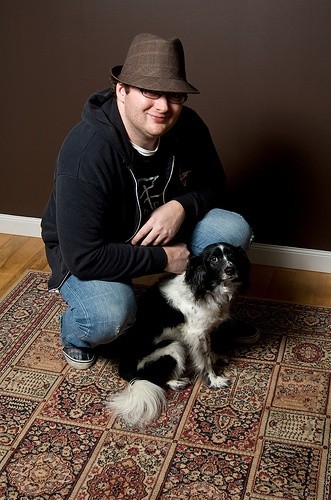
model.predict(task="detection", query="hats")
[110,33,200,94]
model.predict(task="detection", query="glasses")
[135,86,188,104]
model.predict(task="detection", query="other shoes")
[61,345,95,369]
[212,317,261,343]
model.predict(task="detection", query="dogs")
[107,243,249,423]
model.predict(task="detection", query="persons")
[39,33,260,369]
